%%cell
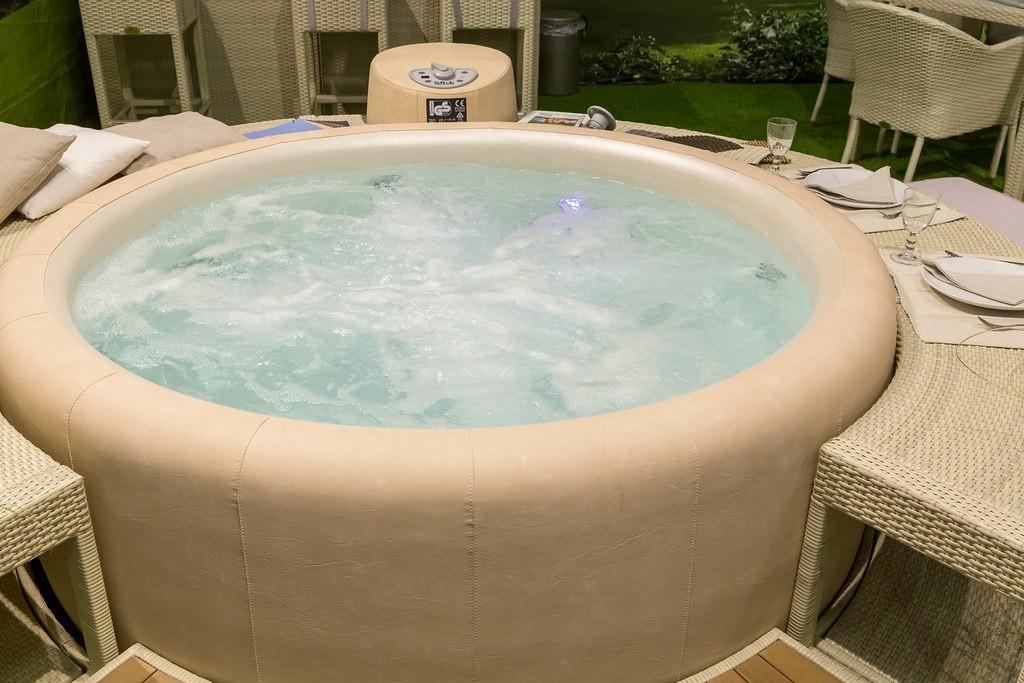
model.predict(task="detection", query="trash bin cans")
[537,8,586,97]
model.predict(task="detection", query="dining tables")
[516,93,1024,683]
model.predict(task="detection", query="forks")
[795,166,853,177]
[874,205,941,221]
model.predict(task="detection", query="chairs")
[835,1,1024,184]
[809,1,890,155]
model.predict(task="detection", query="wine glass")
[890,188,941,265]
[764,117,798,175]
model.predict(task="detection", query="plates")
[804,167,912,209]
[922,253,1023,311]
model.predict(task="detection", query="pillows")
[15,123,148,219]
[0,121,79,234]
[100,109,253,179]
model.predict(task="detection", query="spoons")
[977,316,1024,331]
[944,249,1024,266]
